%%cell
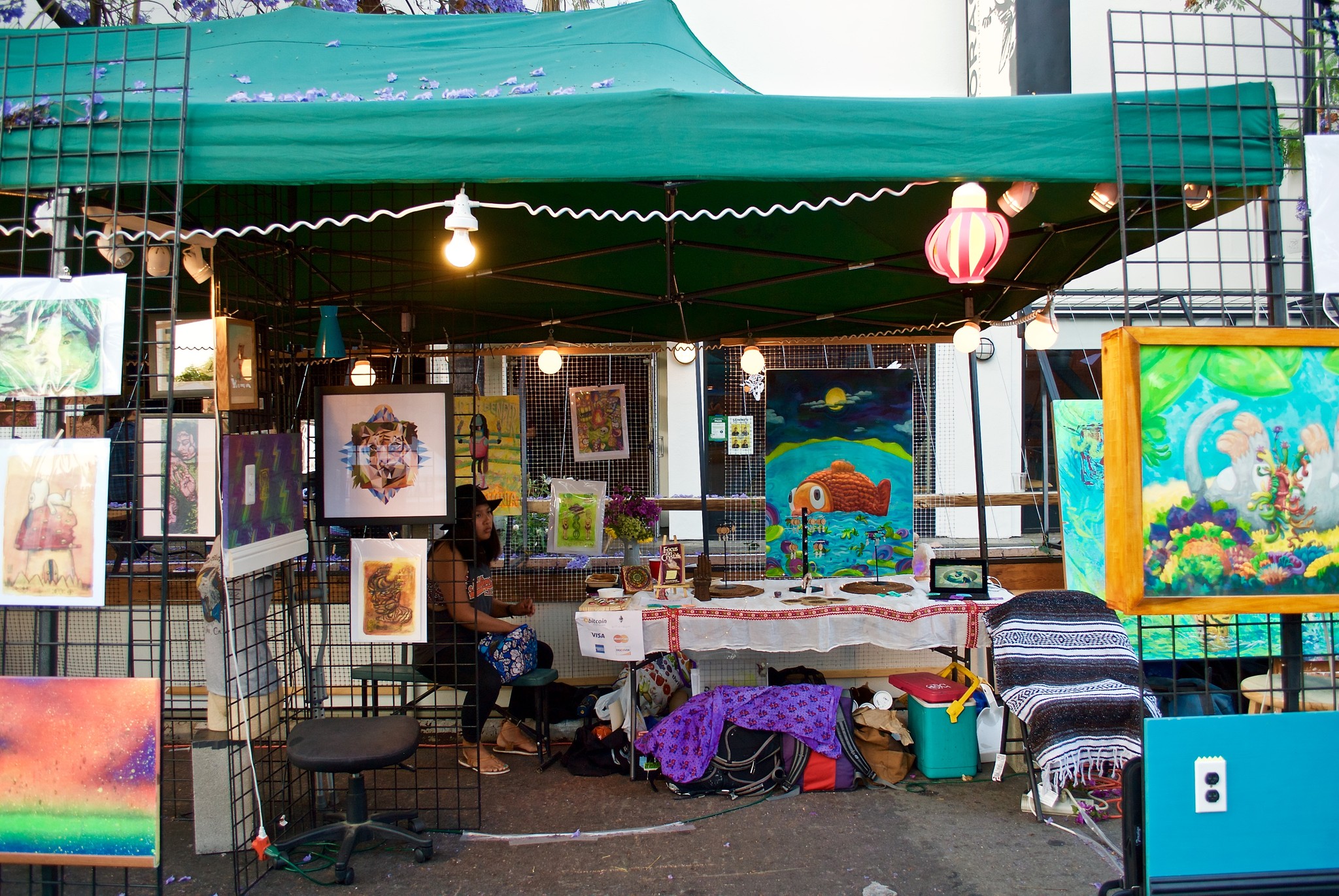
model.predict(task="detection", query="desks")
[575,574,1016,780]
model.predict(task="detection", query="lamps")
[953,313,995,360]
[741,333,765,374]
[998,182,1038,218]
[538,329,562,374]
[1184,184,1212,210]
[97,225,214,284]
[351,345,375,386]
[1024,298,1058,350]
[1089,183,1124,212]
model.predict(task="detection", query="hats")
[440,484,503,531]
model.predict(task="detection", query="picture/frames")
[569,384,630,462]
[138,309,221,542]
[313,384,455,526]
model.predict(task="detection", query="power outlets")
[1195,756,1227,813]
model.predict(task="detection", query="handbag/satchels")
[563,651,916,799]
[478,623,538,684]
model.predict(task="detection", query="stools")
[1241,673,1339,715]
[275,715,433,884]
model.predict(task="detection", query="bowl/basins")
[584,574,618,588]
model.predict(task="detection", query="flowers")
[603,483,662,544]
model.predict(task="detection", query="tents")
[1,4,1308,717]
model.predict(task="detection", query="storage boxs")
[889,662,980,778]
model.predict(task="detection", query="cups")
[597,588,624,598]
[852,700,858,712]
[873,690,892,710]
[859,703,876,710]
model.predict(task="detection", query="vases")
[620,536,642,568]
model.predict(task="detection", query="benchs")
[353,663,562,773]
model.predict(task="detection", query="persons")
[411,482,550,776]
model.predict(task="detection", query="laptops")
[929,558,990,600]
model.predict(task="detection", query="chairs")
[983,590,1162,823]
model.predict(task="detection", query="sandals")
[459,748,510,775]
[493,729,547,756]
